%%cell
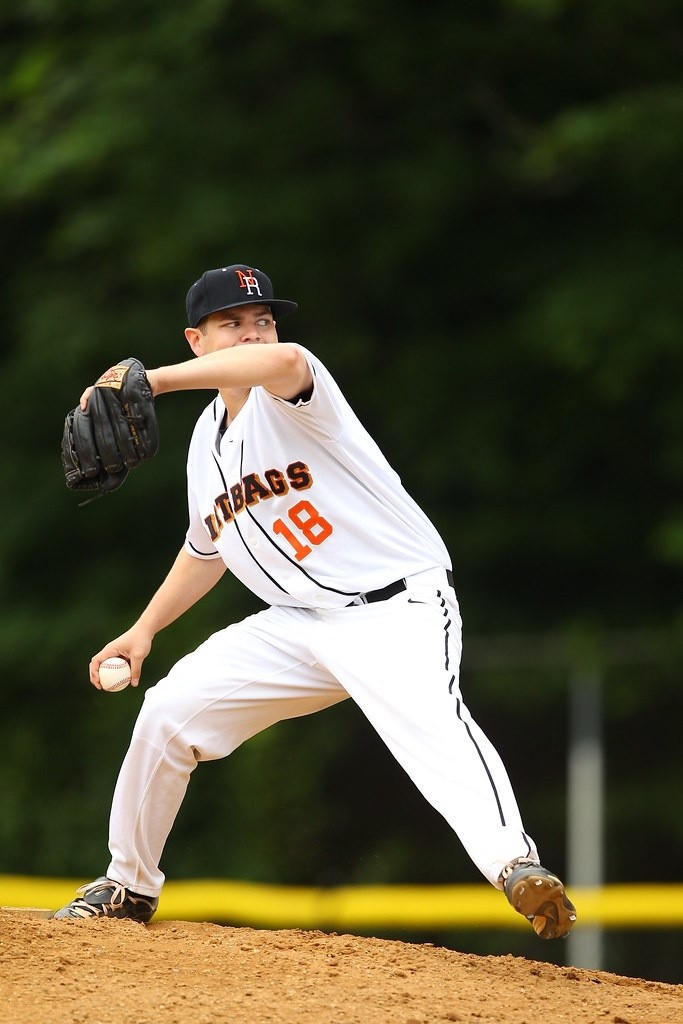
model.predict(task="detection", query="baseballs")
[98,656,132,692]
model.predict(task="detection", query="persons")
[54,266,577,938]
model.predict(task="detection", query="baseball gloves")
[58,357,162,495]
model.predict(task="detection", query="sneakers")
[50,877,158,923]
[497,856,577,940]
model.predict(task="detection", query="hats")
[185,264,299,329]
